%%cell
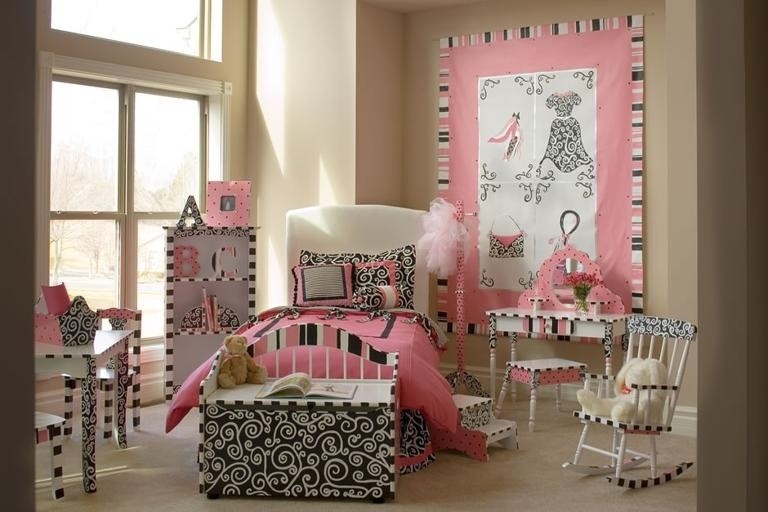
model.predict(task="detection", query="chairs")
[563,313,700,493]
[32,305,142,498]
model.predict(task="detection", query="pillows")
[288,244,417,310]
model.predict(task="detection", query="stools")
[497,356,588,435]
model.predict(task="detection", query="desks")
[486,304,633,419]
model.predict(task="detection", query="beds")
[195,207,437,502]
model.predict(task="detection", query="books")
[199,284,220,331]
[254,372,360,399]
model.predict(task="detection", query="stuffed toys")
[576,356,669,425]
[215,336,268,389]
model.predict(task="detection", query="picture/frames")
[202,182,250,229]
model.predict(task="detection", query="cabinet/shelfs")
[162,220,259,403]
[197,322,404,503]
